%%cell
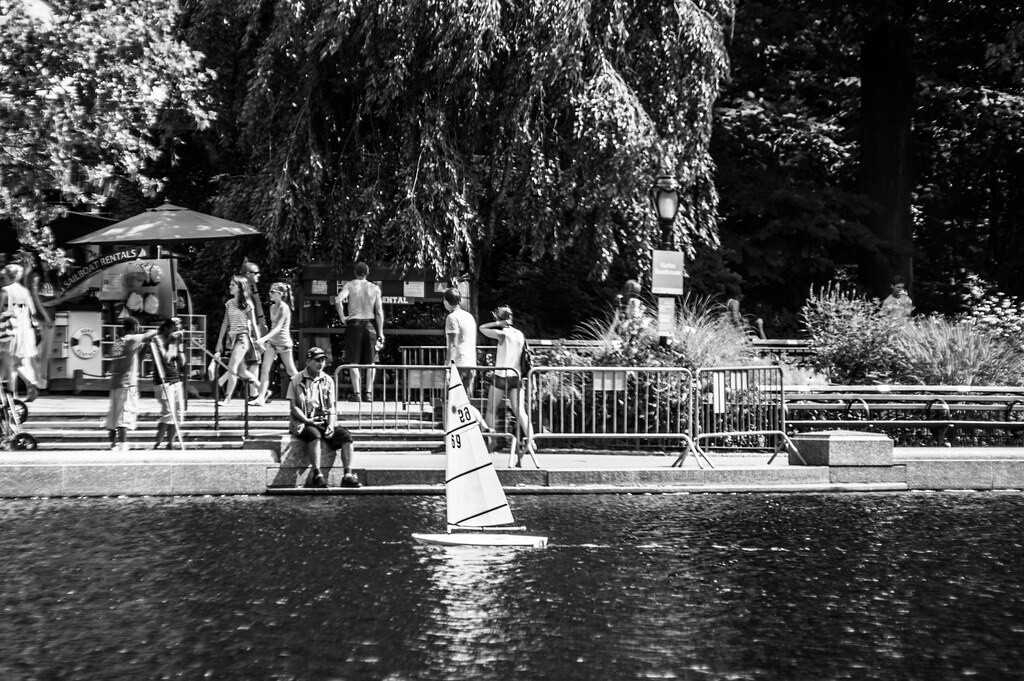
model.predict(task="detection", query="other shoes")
[525,441,537,455]
[247,397,264,405]
[217,395,230,405]
[264,391,272,402]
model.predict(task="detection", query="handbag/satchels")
[208,352,221,381]
[520,340,535,378]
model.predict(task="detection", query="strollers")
[0,371,37,452]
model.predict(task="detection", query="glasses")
[245,271,260,274]
[269,290,280,294]
[169,317,181,322]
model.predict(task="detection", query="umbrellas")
[64,201,264,302]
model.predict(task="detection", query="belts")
[348,319,373,322]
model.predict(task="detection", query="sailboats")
[411,359,550,549]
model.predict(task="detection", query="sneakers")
[362,392,371,402]
[313,473,327,488]
[348,392,360,401]
[341,473,361,487]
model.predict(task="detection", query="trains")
[28,209,214,399]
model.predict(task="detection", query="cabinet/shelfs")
[98,313,206,380]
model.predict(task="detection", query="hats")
[355,262,369,276]
[307,347,328,359]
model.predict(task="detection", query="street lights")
[647,170,681,350]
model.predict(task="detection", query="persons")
[287,347,362,488]
[721,299,767,340]
[215,262,300,406]
[479,307,537,454]
[431,287,477,454]
[880,275,912,319]
[0,239,60,405]
[106,317,186,450]
[621,279,646,338]
[335,262,385,402]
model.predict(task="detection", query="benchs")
[741,383,1024,448]
